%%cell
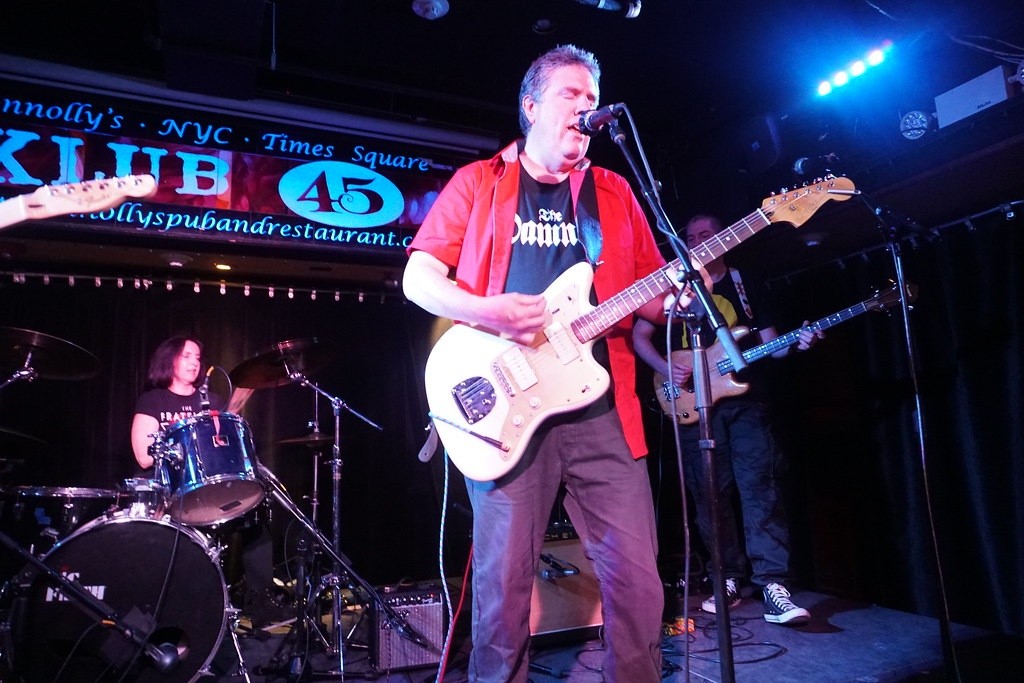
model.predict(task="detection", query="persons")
[632,211,825,623]
[401,44,713,682]
[129,334,282,633]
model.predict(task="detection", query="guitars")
[653,279,916,425]
[424,172,857,482]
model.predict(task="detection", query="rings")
[684,290,691,296]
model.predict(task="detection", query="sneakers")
[763,582,811,623]
[702,578,741,614]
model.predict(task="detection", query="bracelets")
[789,345,797,353]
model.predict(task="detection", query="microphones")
[397,624,422,642]
[538,567,578,583]
[794,153,840,177]
[578,102,620,137]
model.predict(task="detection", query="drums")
[21,503,242,683]
[16,484,128,566]
[152,409,267,524]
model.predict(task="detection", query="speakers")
[371,589,445,676]
[523,530,607,648]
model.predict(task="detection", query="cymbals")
[2,326,98,369]
[228,333,327,389]
[278,432,335,442]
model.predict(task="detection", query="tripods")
[263,375,370,683]
